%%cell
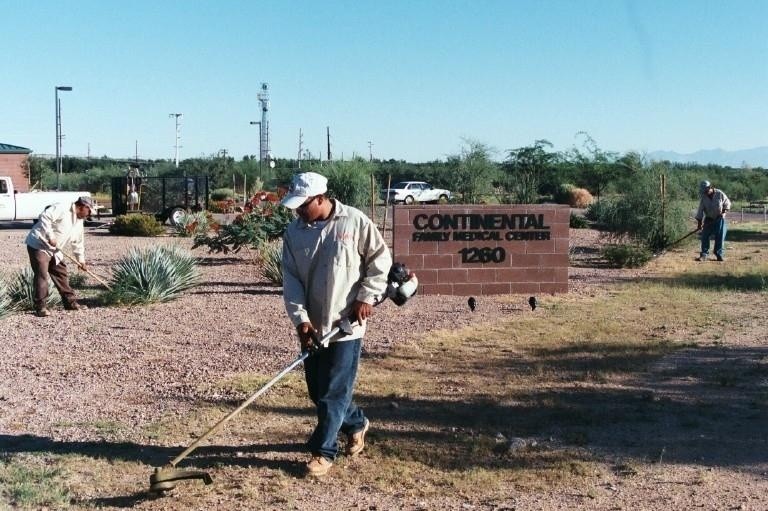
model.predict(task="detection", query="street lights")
[54,86,74,191]
[249,122,264,185]
[170,110,185,170]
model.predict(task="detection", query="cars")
[378,181,453,206]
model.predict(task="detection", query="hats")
[280,170,328,210]
[699,180,711,193]
[78,196,98,217]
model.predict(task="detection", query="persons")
[280,171,393,477]
[23,196,98,316]
[695,180,732,262]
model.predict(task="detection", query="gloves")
[697,220,704,230]
[48,240,58,247]
[77,263,88,273]
[298,321,319,354]
[719,212,726,219]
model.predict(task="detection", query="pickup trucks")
[1,176,95,227]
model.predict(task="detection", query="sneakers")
[345,417,369,456]
[64,302,88,311]
[35,308,51,317]
[716,255,727,262]
[305,452,336,476]
[698,255,708,262]
